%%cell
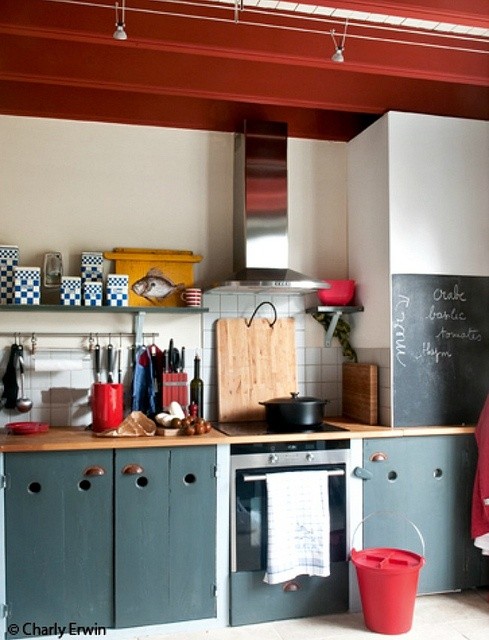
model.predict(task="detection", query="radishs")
[161,416,175,427]
[155,413,167,422]
[168,400,185,419]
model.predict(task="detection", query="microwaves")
[229,438,350,628]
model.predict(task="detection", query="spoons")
[15,345,32,412]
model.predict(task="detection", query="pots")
[258,392,331,430]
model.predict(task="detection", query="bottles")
[190,354,203,420]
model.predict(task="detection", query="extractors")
[203,117,328,295]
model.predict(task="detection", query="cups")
[92,383,123,432]
[180,287,202,307]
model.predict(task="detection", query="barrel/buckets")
[345,510,426,635]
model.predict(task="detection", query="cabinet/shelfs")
[347,111,488,427]
[4,446,216,640]
[362,437,489,593]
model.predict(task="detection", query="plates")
[6,422,49,434]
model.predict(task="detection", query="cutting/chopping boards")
[215,301,298,422]
[342,362,378,425]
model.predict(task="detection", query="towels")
[265,472,330,584]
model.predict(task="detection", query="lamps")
[330,19,349,63]
[113,0,127,40]
